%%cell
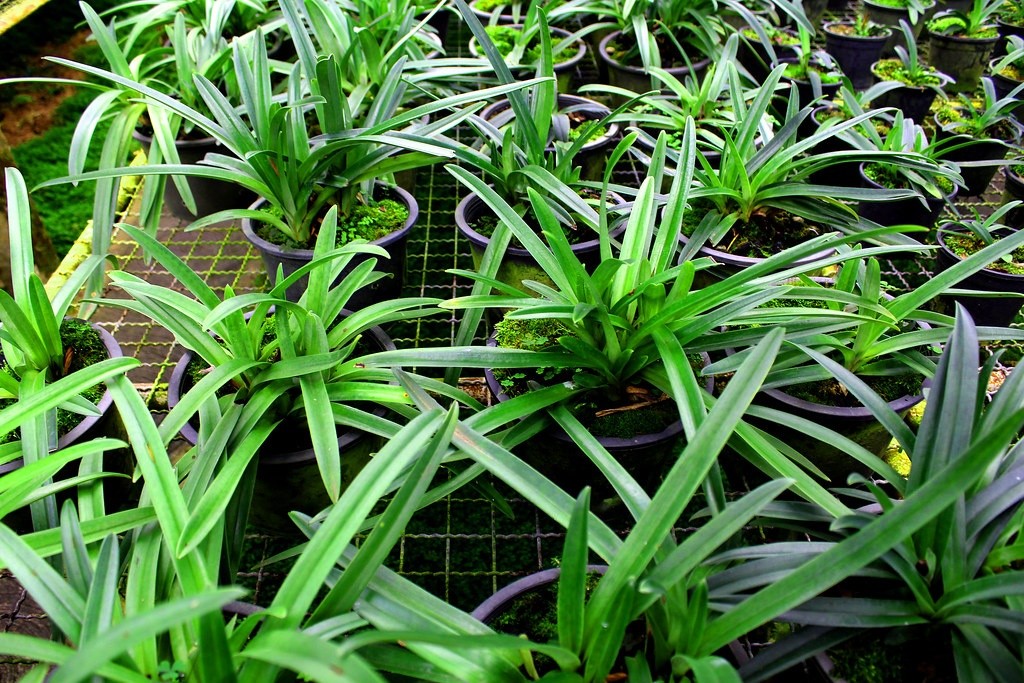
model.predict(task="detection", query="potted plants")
[0,0,1024,683]
